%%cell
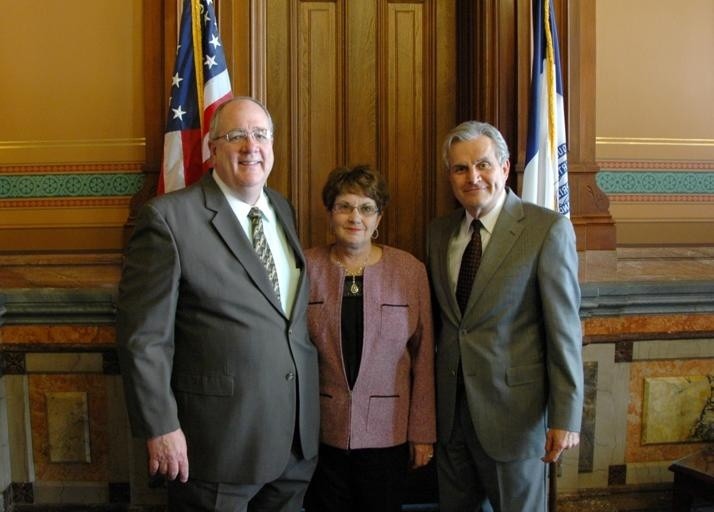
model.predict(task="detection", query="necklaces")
[333,251,376,294]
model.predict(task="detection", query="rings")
[428,455,435,460]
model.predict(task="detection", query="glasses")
[331,203,379,215]
[213,130,270,142]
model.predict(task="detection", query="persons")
[112,96,320,512]
[415,120,584,512]
[302,162,436,512]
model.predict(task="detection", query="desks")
[669,449,713,512]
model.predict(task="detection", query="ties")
[455,220,482,317]
[248,207,281,306]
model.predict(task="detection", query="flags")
[523,1,571,223]
[150,1,241,198]
